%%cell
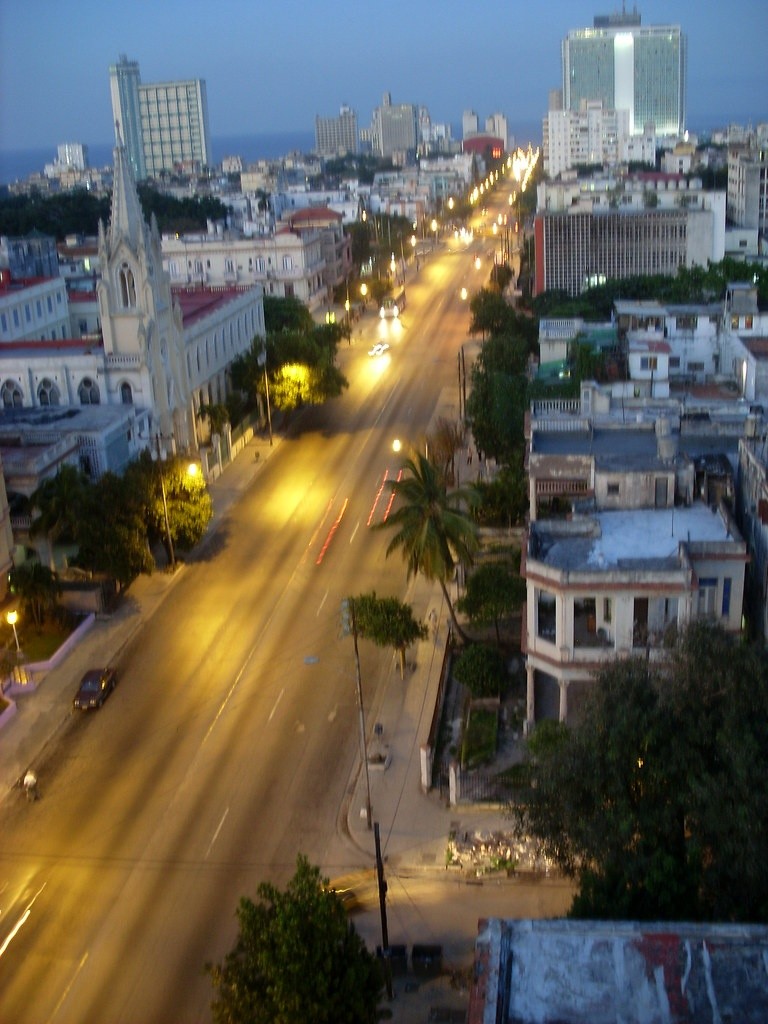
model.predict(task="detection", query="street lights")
[7,611,23,651]
[159,461,198,567]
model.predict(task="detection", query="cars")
[72,668,117,711]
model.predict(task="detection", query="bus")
[377,284,407,318]
